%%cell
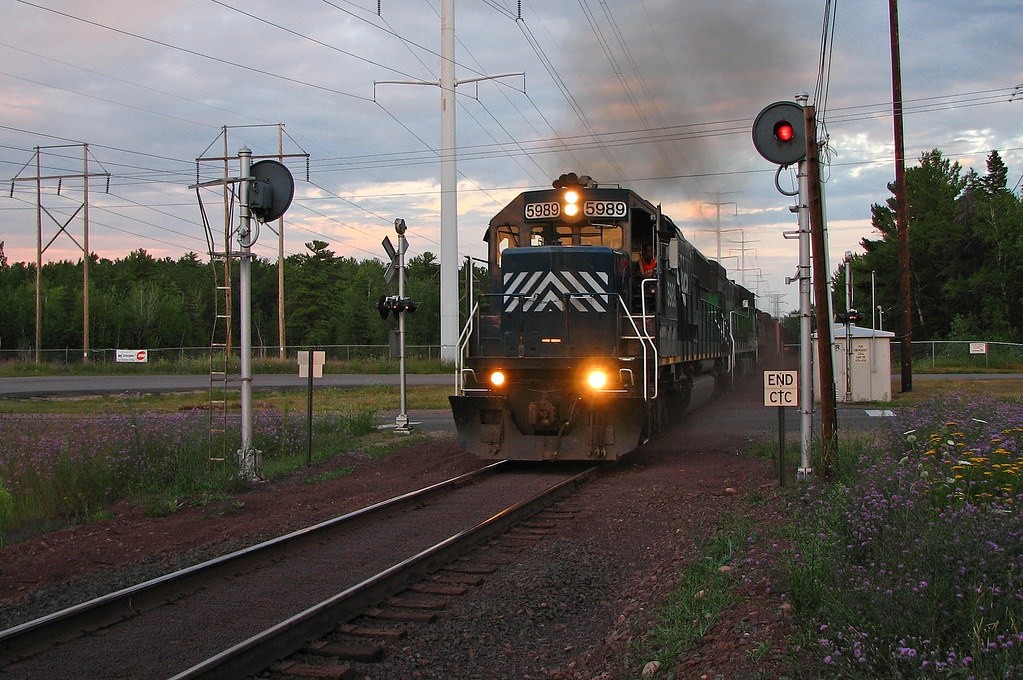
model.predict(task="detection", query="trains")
[447,172,799,461]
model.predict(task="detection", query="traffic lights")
[384,301,417,312]
[752,100,807,164]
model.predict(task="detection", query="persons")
[632,245,657,312]
